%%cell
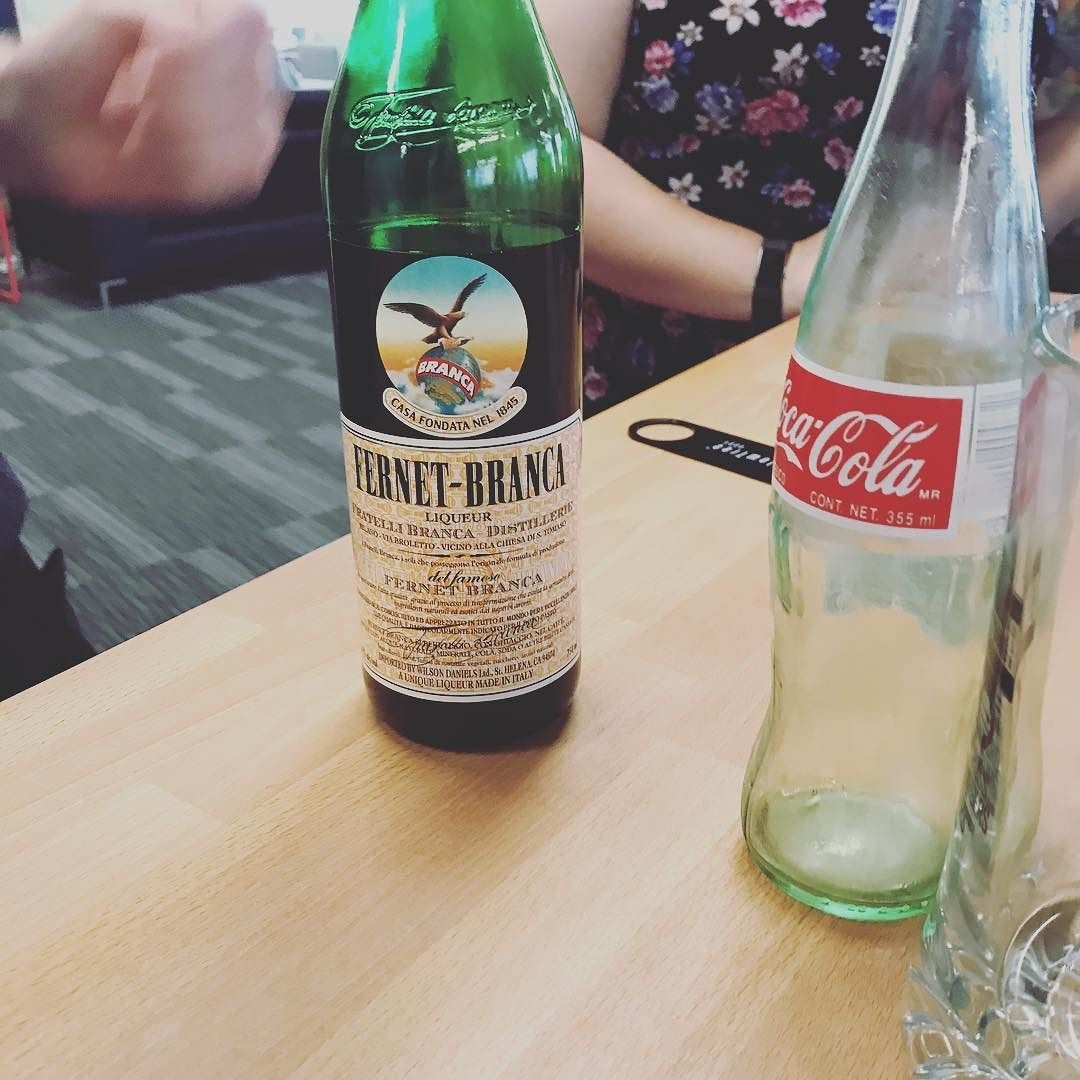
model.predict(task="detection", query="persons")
[529,1,1080,416]
[1,0,297,701]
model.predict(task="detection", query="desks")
[0,292,1080,1080]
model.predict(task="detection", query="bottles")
[739,0,1050,921]
[315,0,587,751]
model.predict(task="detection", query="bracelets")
[751,233,792,326]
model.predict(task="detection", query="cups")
[904,296,1080,1080]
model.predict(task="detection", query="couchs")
[8,90,331,292]
